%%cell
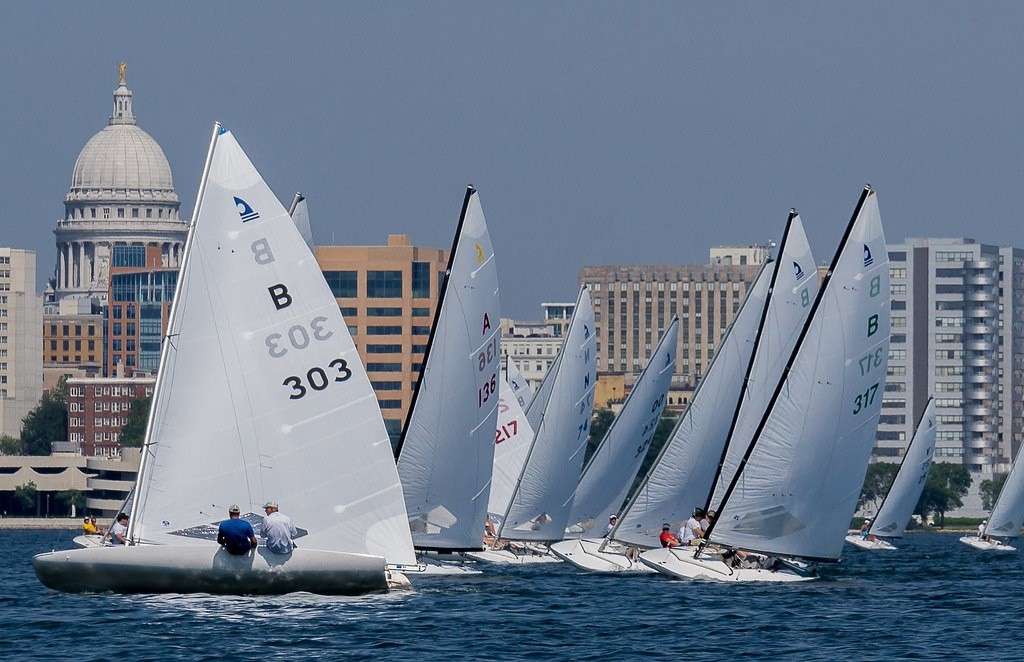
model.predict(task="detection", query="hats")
[262,501,278,509]
[983,520,987,523]
[708,510,716,517]
[229,504,241,513]
[663,523,671,529]
[84,517,90,519]
[610,514,617,518]
[865,520,870,523]
[118,513,130,520]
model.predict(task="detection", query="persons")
[659,507,763,564]
[111,513,129,547]
[259,501,297,555]
[485,517,495,536]
[607,514,618,536]
[977,521,993,543]
[859,520,875,541]
[83,516,102,534]
[218,505,257,556]
[119,62,127,82]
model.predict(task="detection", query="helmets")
[692,507,703,518]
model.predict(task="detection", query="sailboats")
[958,431,1024,552]
[486,286,596,576]
[26,118,419,606]
[557,185,897,584]
[564,314,683,578]
[393,183,504,585]
[846,389,935,554]
